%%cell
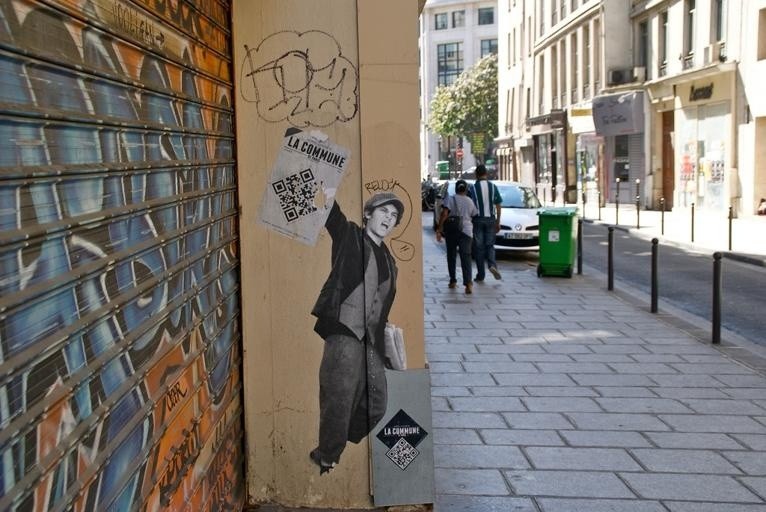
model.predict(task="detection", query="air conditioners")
[608,69,629,85]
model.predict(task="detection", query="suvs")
[456,164,497,180]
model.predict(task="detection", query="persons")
[309,128,403,475]
[435,180,478,294]
[466,164,503,281]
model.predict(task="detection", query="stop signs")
[455,149,464,157]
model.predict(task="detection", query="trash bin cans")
[435,160,449,180]
[536,206,579,278]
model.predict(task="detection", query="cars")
[467,181,546,254]
[431,180,471,235]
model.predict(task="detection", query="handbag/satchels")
[443,215,463,236]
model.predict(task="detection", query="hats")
[365,192,404,224]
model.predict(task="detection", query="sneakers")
[449,282,456,288]
[465,281,473,294]
[489,267,501,280]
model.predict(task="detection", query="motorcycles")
[421,175,438,211]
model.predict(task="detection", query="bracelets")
[436,230,442,233]
